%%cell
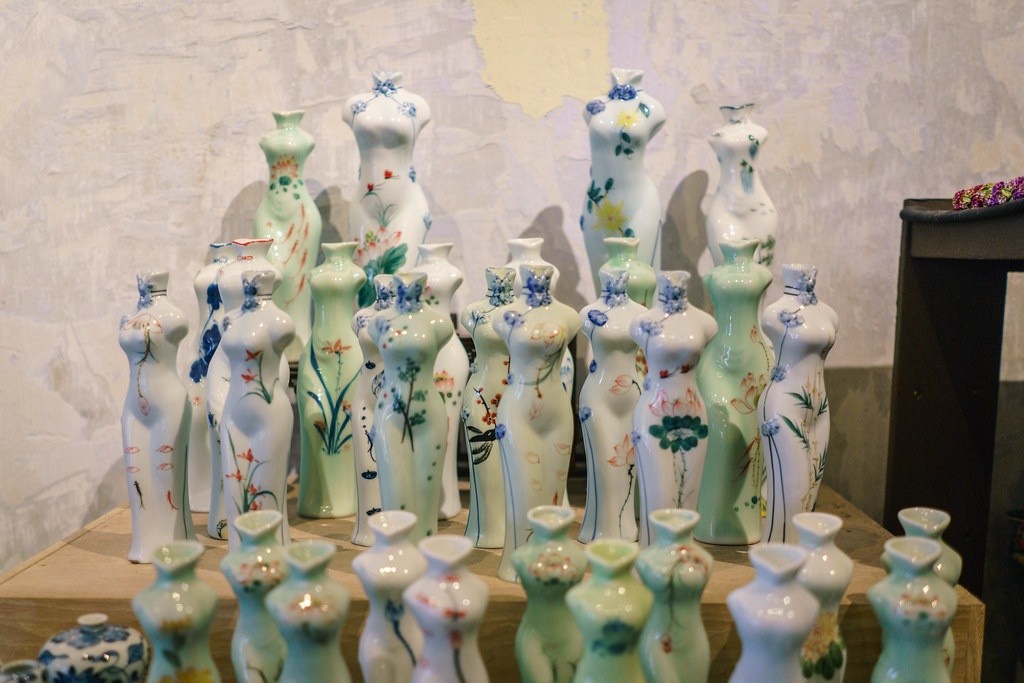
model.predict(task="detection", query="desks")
[882,197,1024,598]
[0,483,986,683]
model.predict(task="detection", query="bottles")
[0,68,962,683]
